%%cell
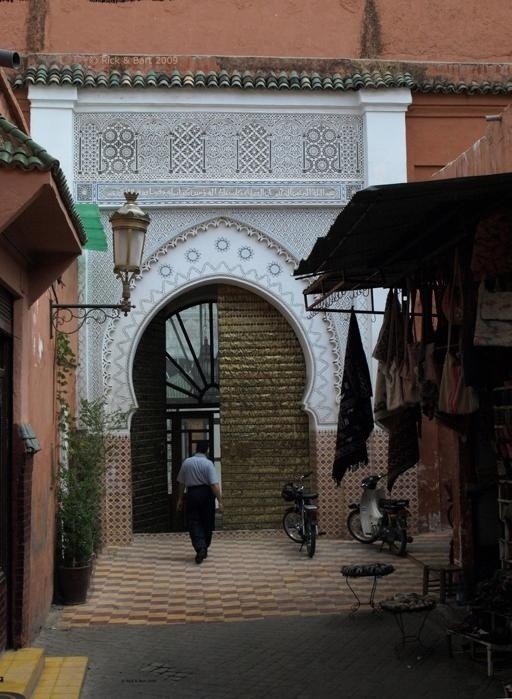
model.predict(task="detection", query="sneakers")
[195,548,207,564]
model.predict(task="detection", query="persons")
[174,438,226,564]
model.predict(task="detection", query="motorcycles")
[347,473,413,556]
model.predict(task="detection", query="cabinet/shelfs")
[447,384,512,677]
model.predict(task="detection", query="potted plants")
[53,457,98,606]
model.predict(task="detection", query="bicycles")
[281,471,326,558]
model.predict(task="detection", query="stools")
[342,564,393,620]
[423,565,462,603]
[379,594,436,661]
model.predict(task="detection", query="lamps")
[49,190,150,337]
